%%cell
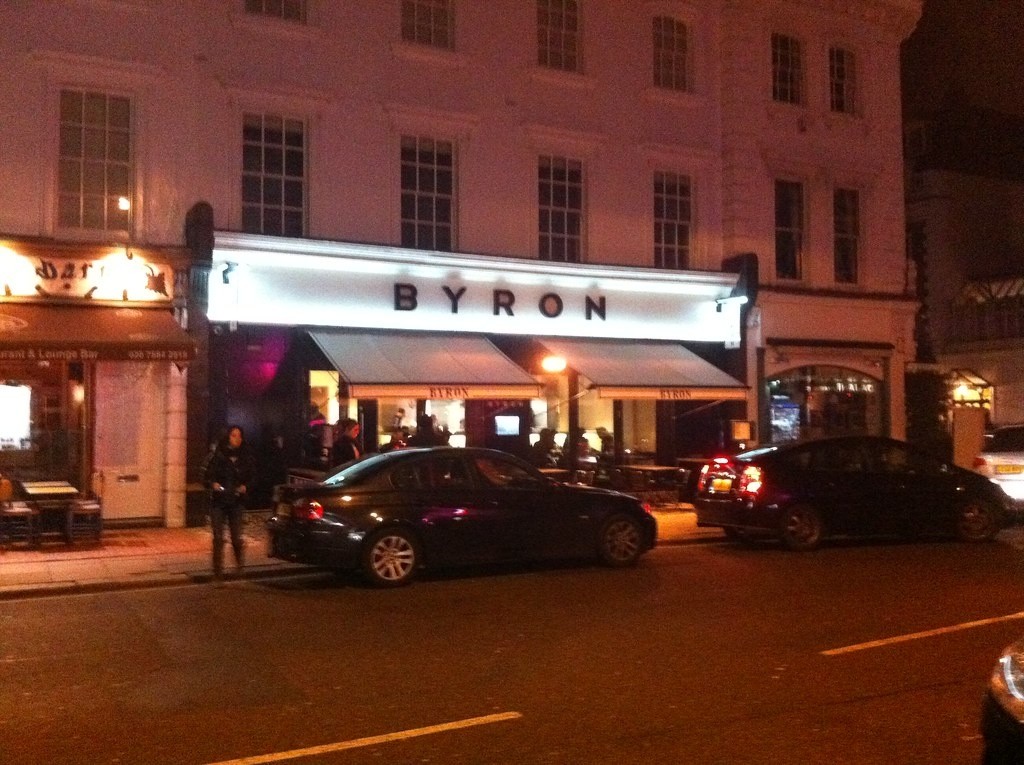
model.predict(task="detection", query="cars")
[693,433,1021,554]
[266,446,658,589]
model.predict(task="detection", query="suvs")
[972,423,1024,507]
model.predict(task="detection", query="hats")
[418,414,431,427]
[540,429,558,437]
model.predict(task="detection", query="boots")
[212,539,226,590]
[231,539,249,581]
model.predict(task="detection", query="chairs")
[450,460,470,484]
[574,470,595,487]
[0,474,43,551]
[661,467,692,513]
[64,471,106,542]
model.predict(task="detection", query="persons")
[597,428,614,452]
[534,427,585,467]
[319,418,360,466]
[378,408,451,453]
[201,424,250,588]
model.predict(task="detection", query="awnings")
[538,339,750,400]
[305,330,540,399]
[0,302,196,359]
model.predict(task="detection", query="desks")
[539,468,571,476]
[619,464,680,508]
[21,479,79,546]
